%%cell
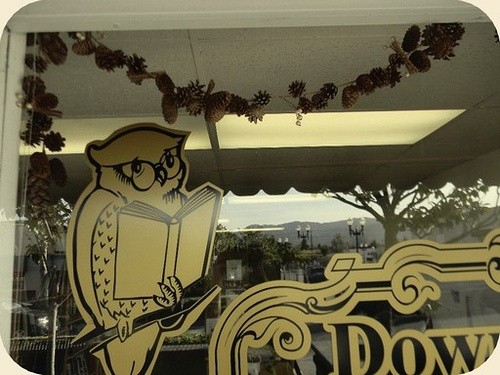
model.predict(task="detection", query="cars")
[10,300,60,343]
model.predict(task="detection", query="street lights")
[346,216,366,250]
[295,224,312,248]
[276,237,289,256]
